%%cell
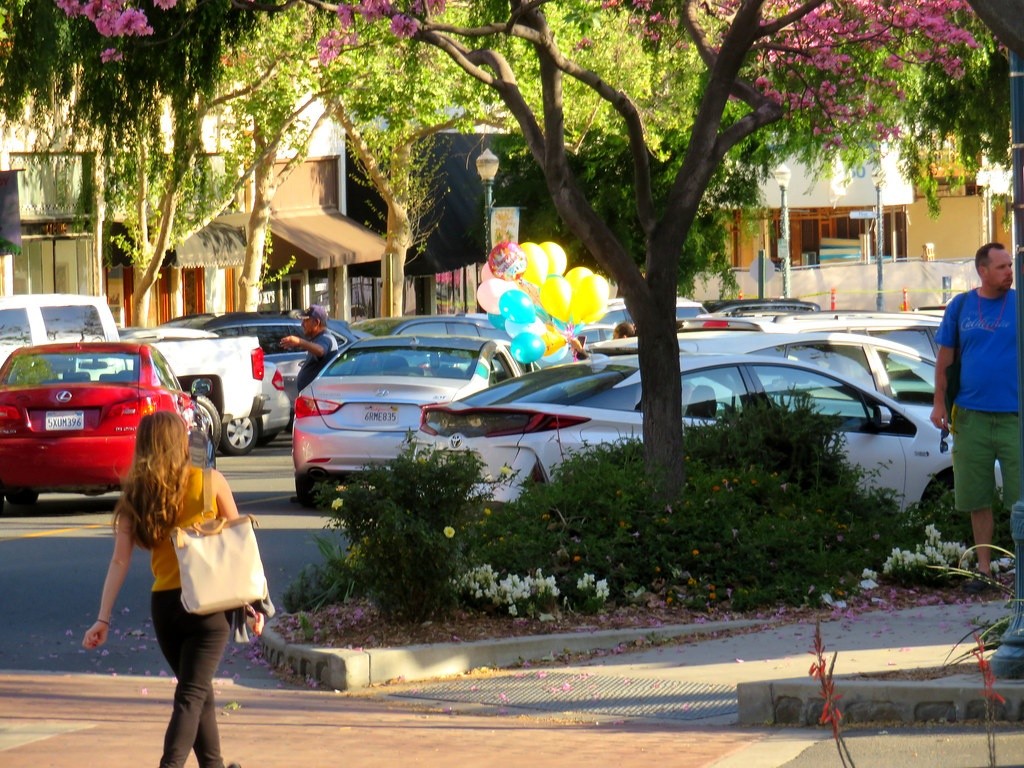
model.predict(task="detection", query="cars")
[589,302,946,416]
[0,344,213,510]
[414,351,1006,533]
[572,295,709,344]
[396,317,574,371]
[119,329,292,457]
[290,333,547,507]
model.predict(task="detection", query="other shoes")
[227,763,241,768]
[964,569,995,593]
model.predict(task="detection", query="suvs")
[201,314,375,411]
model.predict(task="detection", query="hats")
[297,305,327,321]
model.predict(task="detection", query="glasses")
[940,426,958,453]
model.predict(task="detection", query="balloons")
[477,242,610,363]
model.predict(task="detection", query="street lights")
[476,148,500,256]
[873,169,887,311]
[773,165,792,297]
[981,163,994,244]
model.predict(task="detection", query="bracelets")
[96,619,109,625]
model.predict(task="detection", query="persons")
[280,304,338,392]
[81,411,263,768]
[930,242,1020,590]
[571,323,635,360]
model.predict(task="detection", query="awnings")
[107,212,254,267]
[265,213,387,271]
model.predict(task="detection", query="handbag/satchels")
[944,292,963,423]
[171,467,267,614]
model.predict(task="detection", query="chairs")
[685,386,717,419]
[61,372,90,382]
[383,357,410,376]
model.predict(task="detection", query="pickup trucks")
[0,291,271,461]
